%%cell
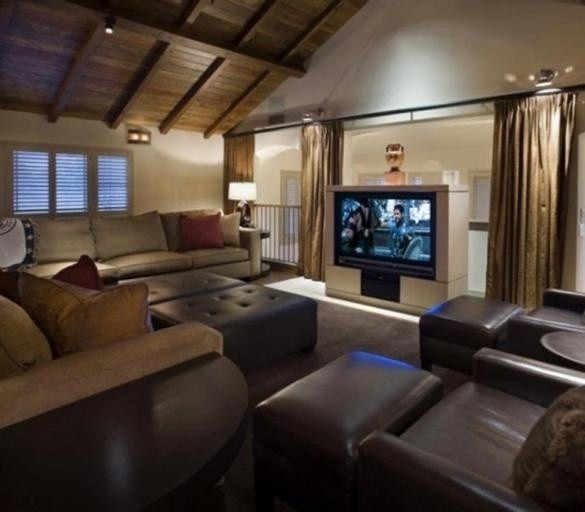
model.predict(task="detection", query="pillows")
[1,216,40,273]
[511,384,585,498]
[177,212,224,250]
[220,211,241,247]
[19,254,153,364]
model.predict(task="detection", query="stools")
[418,295,523,381]
[252,350,443,508]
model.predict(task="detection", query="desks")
[246,229,271,282]
[539,331,585,369]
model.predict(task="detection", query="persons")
[385,206,417,251]
[342,211,364,240]
[358,202,380,244]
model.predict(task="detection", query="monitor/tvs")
[333,190,437,282]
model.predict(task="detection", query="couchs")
[118,272,318,374]
[509,289,585,362]
[0,270,248,510]
[1,207,260,288]
[357,346,585,511]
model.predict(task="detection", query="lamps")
[126,126,151,144]
[227,181,256,227]
[303,108,322,121]
[536,69,555,87]
